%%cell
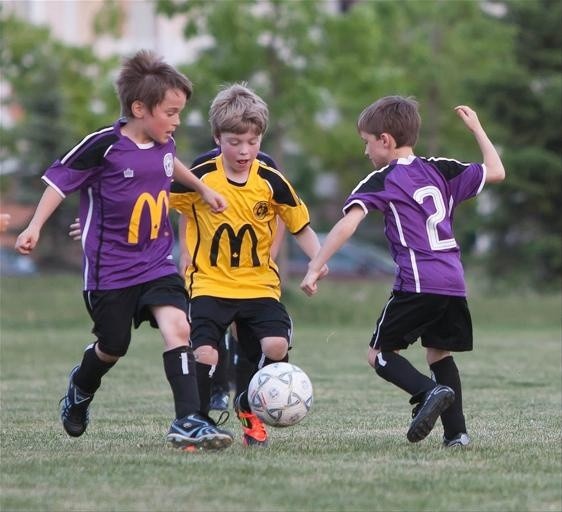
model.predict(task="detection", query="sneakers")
[441,433,471,448]
[406,383,456,444]
[234,391,269,448]
[209,392,231,410]
[60,364,95,438]
[165,412,233,452]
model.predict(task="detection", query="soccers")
[247,362,312,427]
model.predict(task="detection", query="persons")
[298,94,506,450]
[210,321,239,410]
[69,80,328,447]
[14,50,235,448]
